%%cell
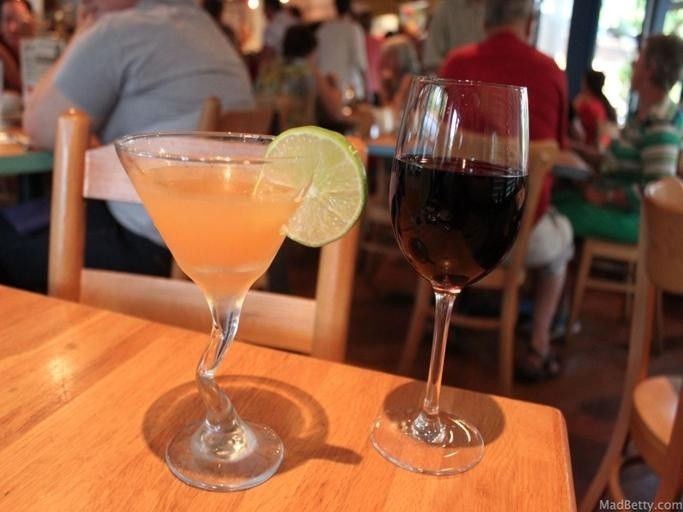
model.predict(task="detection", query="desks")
[0,278,581,510]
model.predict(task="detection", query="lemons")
[253,125,369,249]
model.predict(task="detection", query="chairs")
[44,104,367,362]
[576,174,683,511]
[168,96,281,291]
[324,95,682,378]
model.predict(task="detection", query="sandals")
[517,342,563,382]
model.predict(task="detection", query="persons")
[517,34,682,343]
[0,0,38,93]
[257,0,426,137]
[437,2,575,383]
[1,2,259,293]
[570,72,619,151]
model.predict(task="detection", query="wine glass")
[113,130,315,491]
[363,77,532,478]
